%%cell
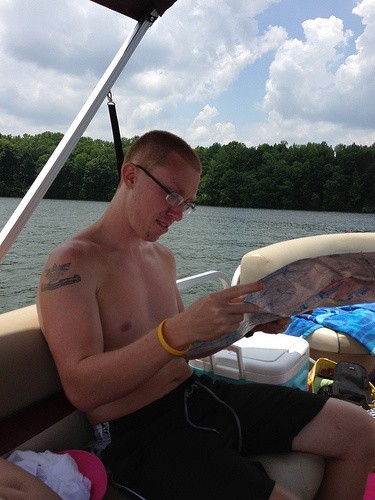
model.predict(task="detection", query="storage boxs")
[187,330,310,392]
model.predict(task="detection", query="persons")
[0,457,63,500]
[36,130,375,500]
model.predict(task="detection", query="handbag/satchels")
[317,361,372,410]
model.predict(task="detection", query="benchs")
[0,232,374,500]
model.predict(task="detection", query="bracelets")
[245,332,253,339]
[158,318,192,357]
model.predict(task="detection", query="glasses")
[135,164,197,216]
